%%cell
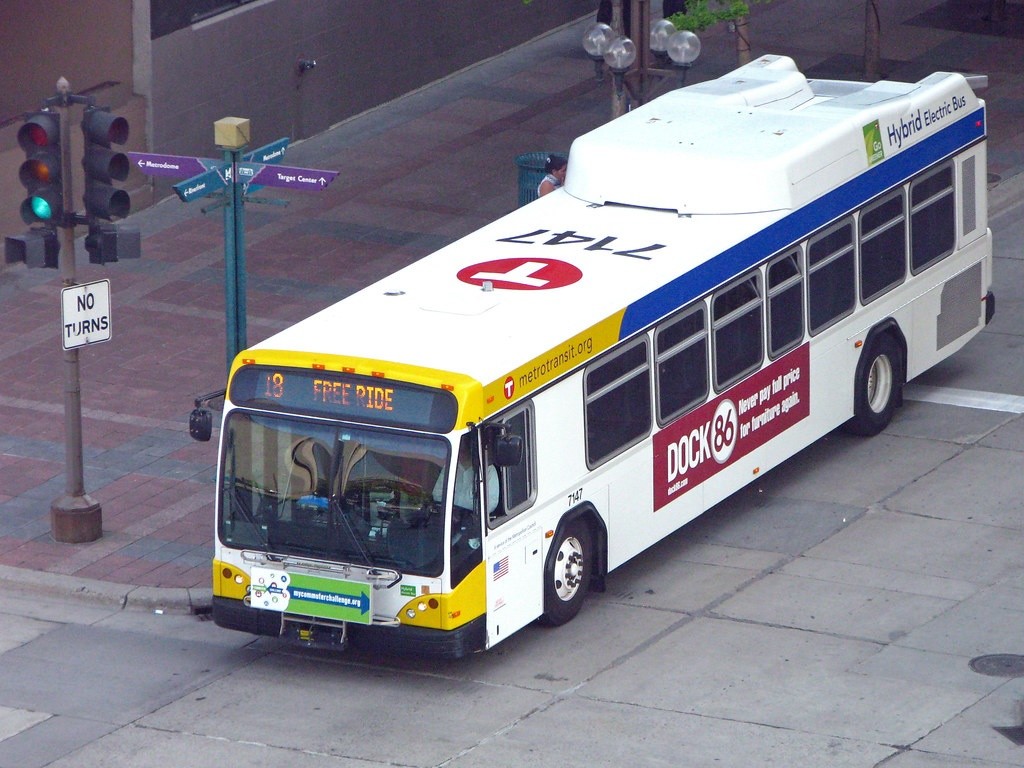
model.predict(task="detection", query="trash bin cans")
[512,151,569,208]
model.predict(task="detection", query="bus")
[189,54,996,671]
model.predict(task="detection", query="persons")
[432,433,499,515]
[536,157,567,199]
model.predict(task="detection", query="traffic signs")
[128,152,223,177]
[236,163,341,193]
[173,163,231,203]
[240,138,291,164]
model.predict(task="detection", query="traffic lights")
[84,110,131,224]
[17,111,63,224]
